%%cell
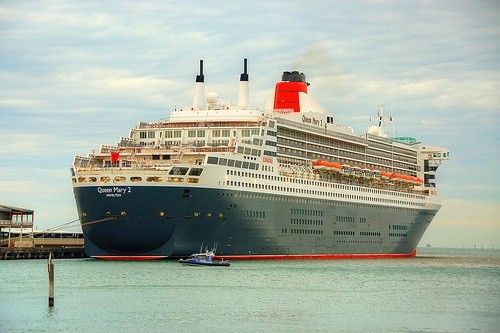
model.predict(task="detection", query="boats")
[174,254,232,266]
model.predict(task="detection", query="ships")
[73,60,451,258]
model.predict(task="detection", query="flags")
[111,152,119,163]
